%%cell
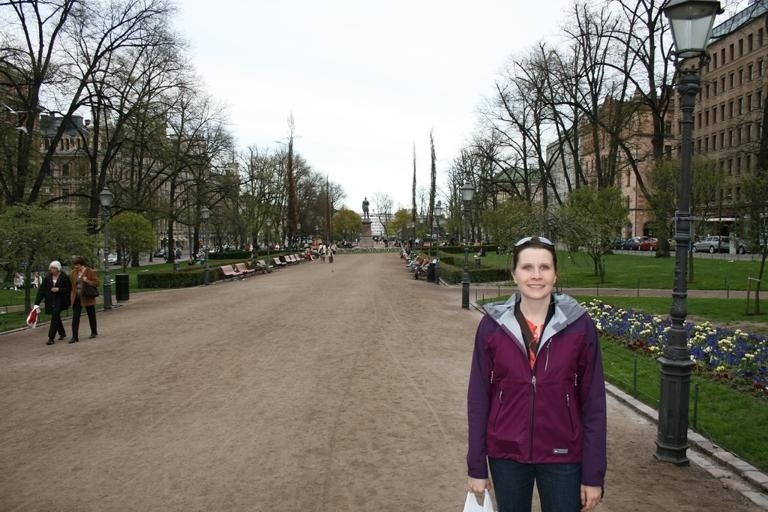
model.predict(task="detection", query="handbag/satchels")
[81,282,99,297]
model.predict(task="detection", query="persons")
[176,247,181,259]
[69,256,100,343]
[361,196,369,218]
[400,247,436,280]
[257,262,272,273]
[249,251,257,267]
[31,272,42,288]
[197,250,205,264]
[34,260,72,345]
[467,236,606,511]
[305,242,337,272]
[14,273,24,289]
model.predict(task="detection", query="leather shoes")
[58,334,66,340]
[47,340,54,344]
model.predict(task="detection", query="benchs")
[218,250,319,285]
[404,255,433,281]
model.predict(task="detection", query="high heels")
[68,337,79,343]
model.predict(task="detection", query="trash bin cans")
[115,275,129,299]
[427,263,436,282]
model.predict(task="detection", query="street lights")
[653,0,725,468]
[295,220,301,253]
[460,170,475,309]
[265,216,273,273]
[396,212,426,273]
[431,200,443,284]
[201,203,211,285]
[98,186,115,310]
[313,224,319,250]
[342,227,361,243]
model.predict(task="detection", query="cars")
[692,235,730,253]
[13,257,49,271]
[585,236,677,252]
[737,233,768,255]
[154,247,182,258]
[102,251,130,266]
[200,235,314,254]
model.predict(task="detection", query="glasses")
[515,237,555,248]
